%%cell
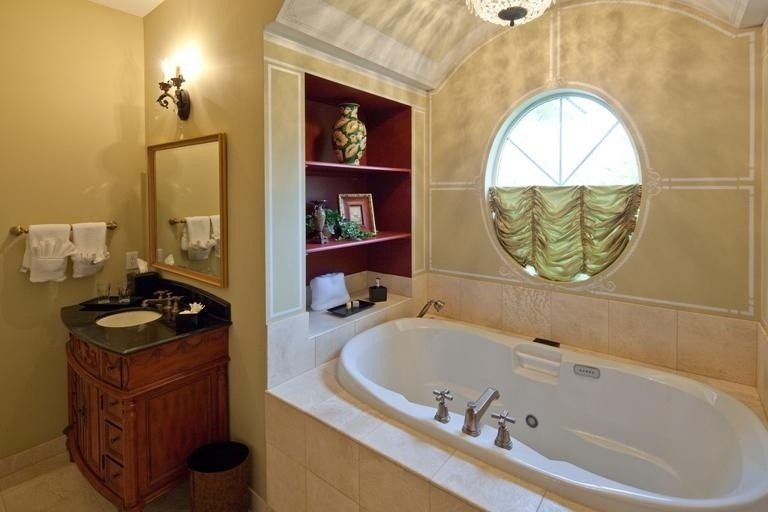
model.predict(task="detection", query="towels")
[19,224,77,283]
[71,222,110,279]
[210,214,220,257]
[180,216,216,260]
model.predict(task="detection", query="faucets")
[460,386,500,437]
[141,291,172,311]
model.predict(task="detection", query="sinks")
[94,307,163,327]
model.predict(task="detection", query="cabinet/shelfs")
[62,322,230,512]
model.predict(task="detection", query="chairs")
[303,72,411,336]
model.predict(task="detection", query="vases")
[332,103,367,165]
[310,200,328,244]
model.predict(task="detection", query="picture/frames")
[338,193,376,236]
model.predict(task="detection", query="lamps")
[155,66,191,121]
[465,0,555,28]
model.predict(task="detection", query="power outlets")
[126,251,139,269]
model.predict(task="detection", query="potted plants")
[305,207,373,240]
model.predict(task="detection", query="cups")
[117,283,131,303]
[98,283,112,304]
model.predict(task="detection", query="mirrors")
[147,132,229,289]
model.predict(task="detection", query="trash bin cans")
[188,441,252,511]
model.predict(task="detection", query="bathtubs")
[336,315,767,512]
